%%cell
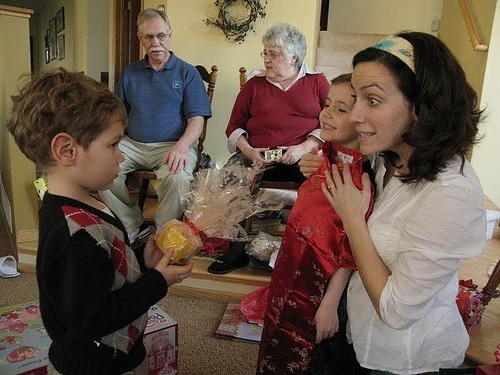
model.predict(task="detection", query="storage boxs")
[0,300,179,375]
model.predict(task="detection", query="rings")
[327,185,334,190]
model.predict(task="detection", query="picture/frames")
[44,6,65,64]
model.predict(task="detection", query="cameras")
[265,149,283,161]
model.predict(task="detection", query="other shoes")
[134,222,151,240]
[207,245,249,275]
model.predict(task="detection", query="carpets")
[0,269,262,375]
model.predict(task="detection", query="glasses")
[260,51,283,59]
[139,33,169,43]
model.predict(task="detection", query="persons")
[7,67,196,375]
[208,24,488,375]
[95,9,211,243]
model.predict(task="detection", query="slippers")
[0,254,21,278]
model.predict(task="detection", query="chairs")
[239,67,303,202]
[123,64,217,212]
